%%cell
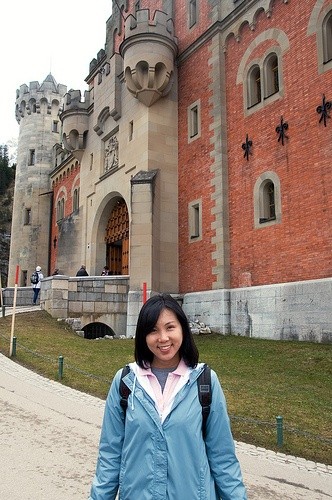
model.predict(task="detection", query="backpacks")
[30,271,39,284]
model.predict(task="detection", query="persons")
[91,296,247,500]
[52,268,60,276]
[30,265,44,305]
[76,265,89,275]
[100,265,112,276]
